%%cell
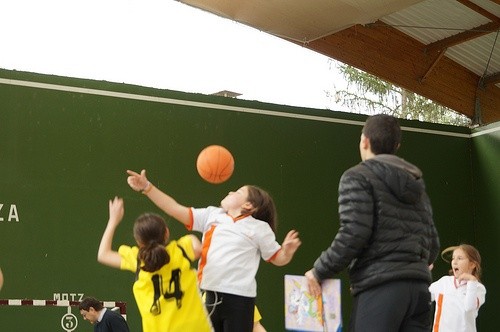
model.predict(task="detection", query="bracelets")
[142,182,153,194]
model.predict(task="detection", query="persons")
[126,169,302,332]
[79,297,130,332]
[428,244,487,332]
[304,113,441,332]
[97,195,215,332]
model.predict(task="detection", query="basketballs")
[196,146,234,184]
